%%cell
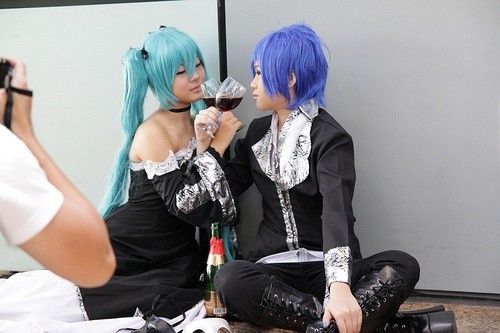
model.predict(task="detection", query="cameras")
[0,58,14,89]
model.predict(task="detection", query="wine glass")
[195,77,248,139]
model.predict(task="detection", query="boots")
[256,265,457,333]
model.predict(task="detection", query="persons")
[66,24,245,322]
[196,24,459,333]
[0,56,120,333]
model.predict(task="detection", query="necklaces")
[169,105,191,113]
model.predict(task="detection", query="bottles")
[204,223,227,322]
